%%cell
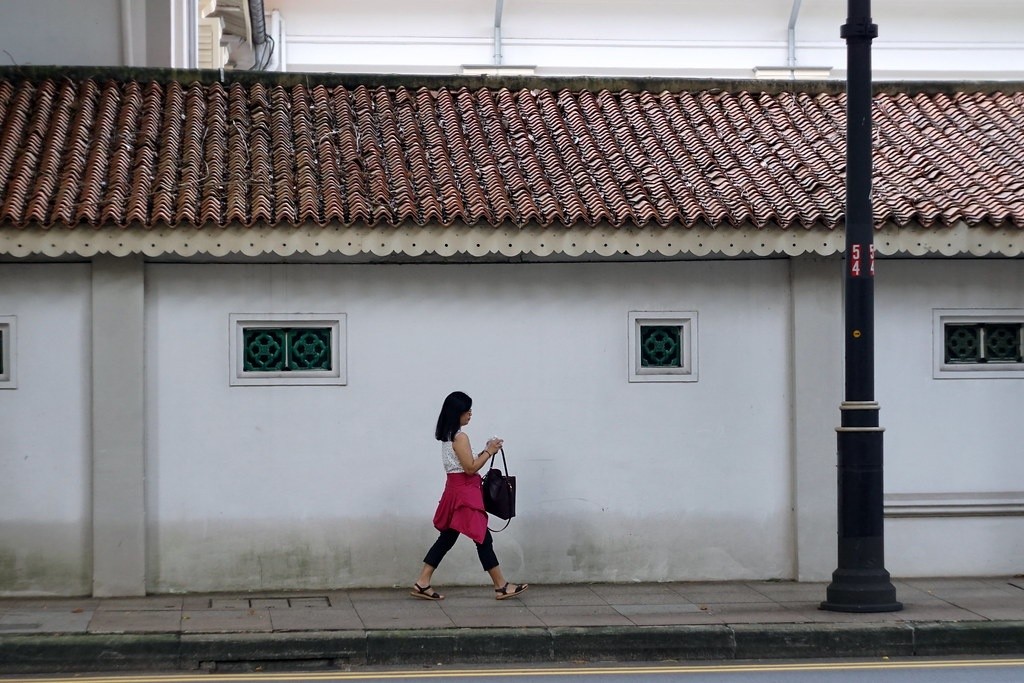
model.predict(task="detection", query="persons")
[409,391,529,600]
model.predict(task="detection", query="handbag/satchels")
[481,448,516,520]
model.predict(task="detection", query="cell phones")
[492,436,497,440]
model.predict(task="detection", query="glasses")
[465,409,471,413]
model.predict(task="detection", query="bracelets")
[485,451,491,456]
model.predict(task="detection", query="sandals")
[495,582,528,600]
[410,583,445,600]
